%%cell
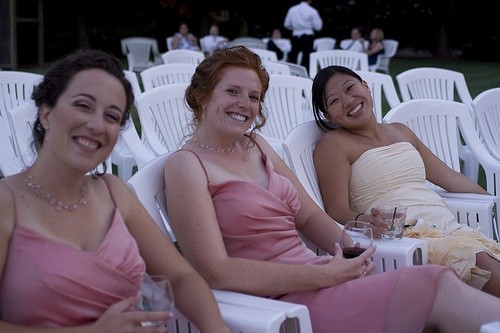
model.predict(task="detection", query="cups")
[374,201,407,240]
[339,221,374,259]
[133,275,174,327]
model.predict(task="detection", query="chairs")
[0,36,500,333]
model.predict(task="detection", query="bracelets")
[354,212,365,229]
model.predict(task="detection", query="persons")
[312,65,500,297]
[363,29,385,65]
[0,50,231,333]
[284,0,323,75]
[272,29,281,38]
[343,28,368,51]
[165,46,500,333]
[172,24,200,50]
[203,26,228,49]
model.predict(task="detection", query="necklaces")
[21,166,88,212]
[186,137,237,154]
